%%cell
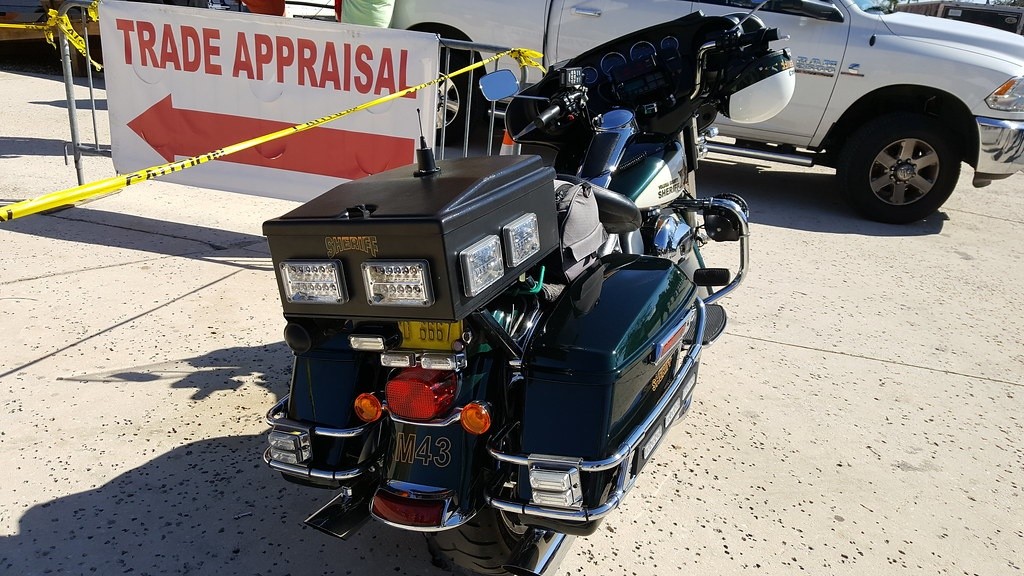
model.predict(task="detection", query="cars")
[385,1,1024,225]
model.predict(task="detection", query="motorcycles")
[263,0,855,575]
